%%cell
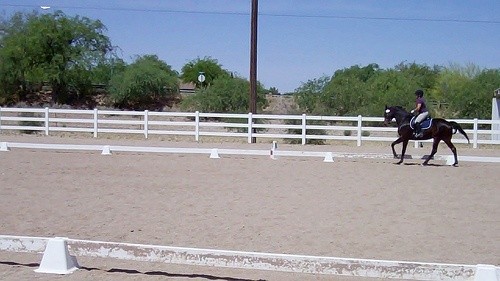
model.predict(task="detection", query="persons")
[411,90,429,137]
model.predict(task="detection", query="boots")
[413,122,421,135]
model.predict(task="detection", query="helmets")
[415,90,423,97]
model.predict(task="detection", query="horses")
[382,104,472,168]
